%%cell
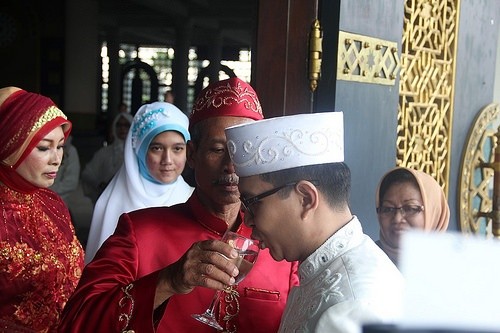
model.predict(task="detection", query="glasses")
[376,205,425,217]
[239,180,320,217]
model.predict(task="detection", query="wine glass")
[190,231,260,329]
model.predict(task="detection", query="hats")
[225,111,344,176]
[188,78,264,130]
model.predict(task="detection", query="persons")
[374,167,451,269]
[83,100,195,268]
[58,81,302,332]
[0,85,89,332]
[224,113,402,332]
[49,88,182,231]
